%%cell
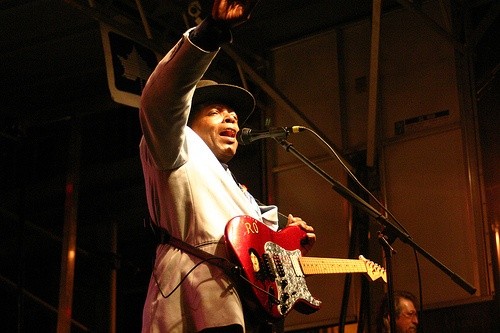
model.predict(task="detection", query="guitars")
[221,215,388,324]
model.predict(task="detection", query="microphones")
[236,125,306,145]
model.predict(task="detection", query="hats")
[189,80,255,121]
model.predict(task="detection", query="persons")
[138,0,317,333]
[380,290,419,333]
